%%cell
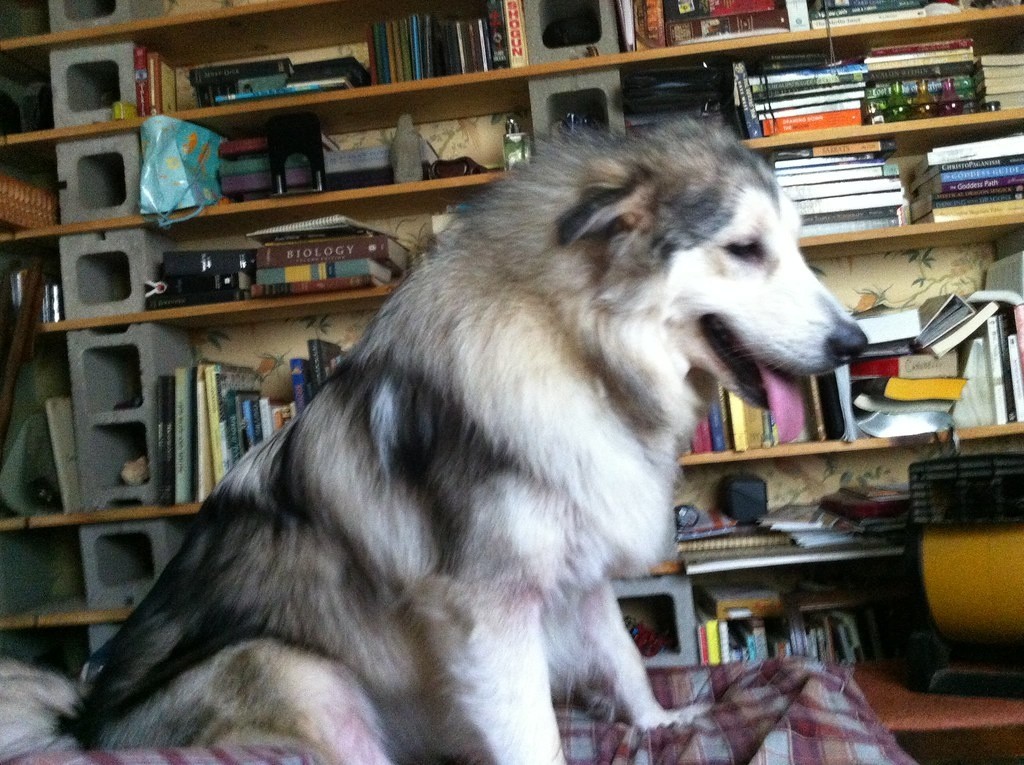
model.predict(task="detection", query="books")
[11,0,1024,667]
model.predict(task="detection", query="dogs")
[0,111,866,764]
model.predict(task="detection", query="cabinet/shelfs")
[0,0,1024,670]
